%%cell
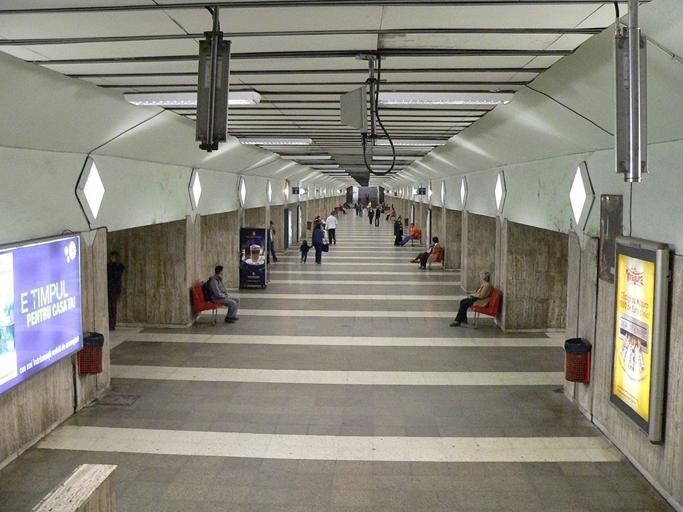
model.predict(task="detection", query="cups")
[250,247,259,262]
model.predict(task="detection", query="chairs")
[428,247,444,270]
[471,288,502,329]
[411,229,420,247]
[189,279,222,327]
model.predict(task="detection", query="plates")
[246,259,264,266]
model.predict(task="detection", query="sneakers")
[273,259,279,263]
[299,260,306,264]
[410,259,420,264]
[225,316,239,324]
[418,266,426,270]
[329,242,336,245]
[449,321,468,327]
[315,260,321,264]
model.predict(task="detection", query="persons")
[298,212,337,265]
[410,236,434,263]
[393,215,404,246]
[337,197,396,227]
[207,264,240,323]
[105,249,125,332]
[447,270,492,328]
[268,220,280,262]
[397,223,420,246]
[414,236,441,270]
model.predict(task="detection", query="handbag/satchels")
[322,243,329,252]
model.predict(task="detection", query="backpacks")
[203,280,212,301]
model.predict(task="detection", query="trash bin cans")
[565,337,592,385]
[76,332,104,376]
[335,207,338,213]
[307,222,312,229]
[405,218,408,226]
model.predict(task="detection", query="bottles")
[241,249,245,260]
